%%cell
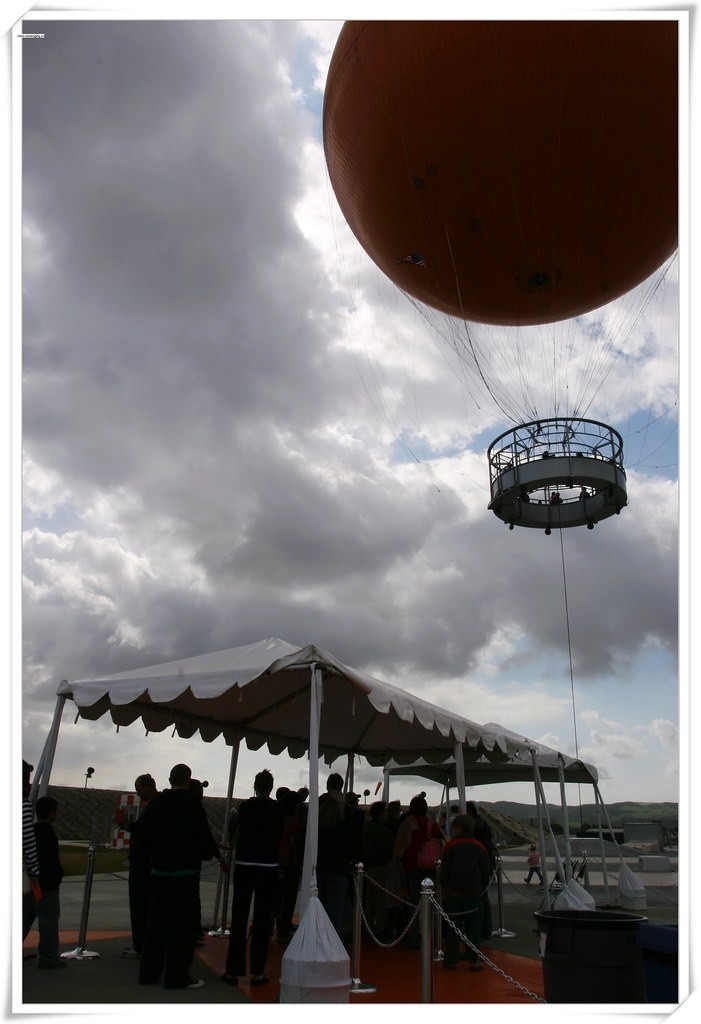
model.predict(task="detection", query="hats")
[134,774,155,793]
[346,791,361,801]
[23,761,33,772]
[190,779,209,796]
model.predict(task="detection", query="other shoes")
[187,979,206,989]
[251,975,270,987]
[471,965,485,971]
[221,975,239,986]
[524,878,530,883]
[121,952,141,958]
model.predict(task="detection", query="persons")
[550,492,562,504]
[23,759,65,971]
[542,451,549,460]
[524,846,543,885]
[579,487,590,500]
[577,452,583,457]
[119,764,494,991]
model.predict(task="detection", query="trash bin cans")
[534,910,650,1002]
[647,923,678,1003]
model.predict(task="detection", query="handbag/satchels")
[411,815,442,878]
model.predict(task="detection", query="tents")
[30,638,610,919]
